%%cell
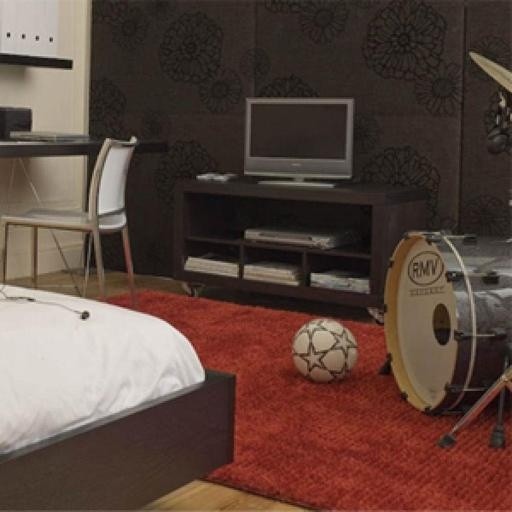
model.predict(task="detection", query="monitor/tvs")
[244,97,353,187]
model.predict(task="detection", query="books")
[182,254,371,296]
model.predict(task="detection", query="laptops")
[10,131,91,143]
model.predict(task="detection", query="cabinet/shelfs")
[178,181,428,323]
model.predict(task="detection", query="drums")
[384,231,512,414]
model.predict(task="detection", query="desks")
[0,138,165,157]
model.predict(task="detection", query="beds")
[0,281,237,512]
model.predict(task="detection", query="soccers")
[292,319,357,382]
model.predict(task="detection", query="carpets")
[92,287,512,512]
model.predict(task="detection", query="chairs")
[0,136,139,309]
